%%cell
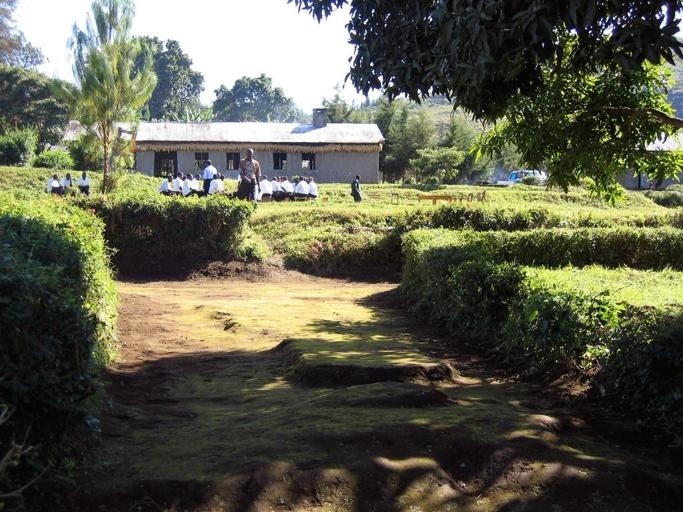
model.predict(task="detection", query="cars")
[508,170,549,185]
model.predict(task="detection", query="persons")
[202,159,217,192]
[60,172,77,198]
[77,171,90,195]
[237,147,262,202]
[47,174,62,195]
[350,174,362,203]
[156,172,318,202]
